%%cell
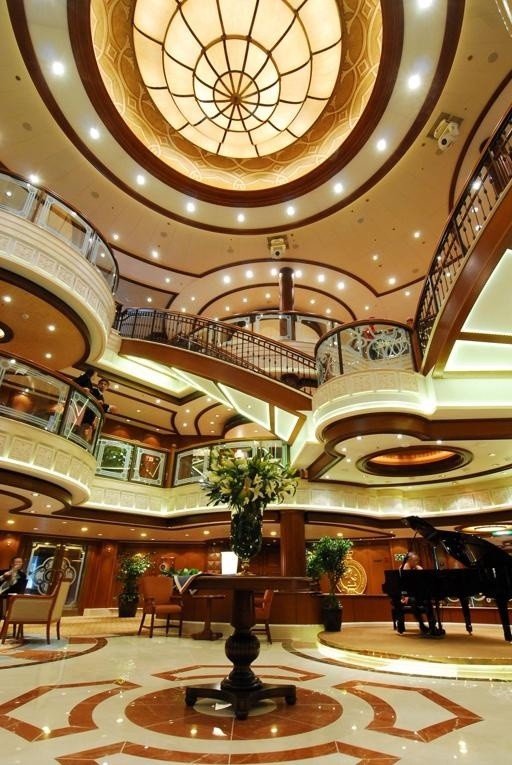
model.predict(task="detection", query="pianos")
[380,515,512,643]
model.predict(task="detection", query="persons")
[398,552,439,637]
[1,557,26,621]
[42,367,111,449]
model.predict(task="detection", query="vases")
[229,506,264,576]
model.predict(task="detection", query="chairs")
[0,575,74,644]
[137,592,184,639]
[111,552,153,618]
[307,534,353,632]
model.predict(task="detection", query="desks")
[167,571,319,722]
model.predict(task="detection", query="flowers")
[184,441,304,516]
